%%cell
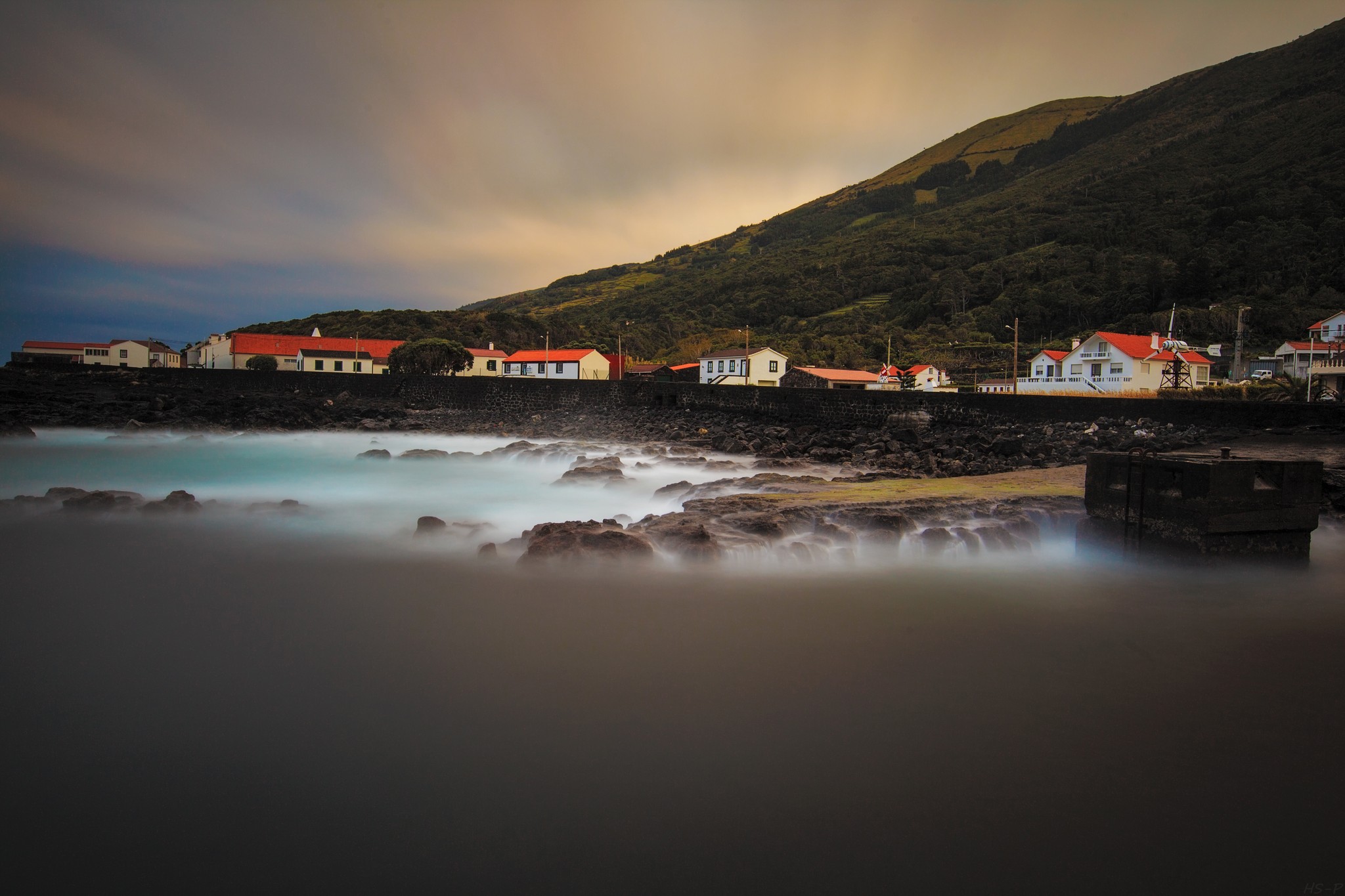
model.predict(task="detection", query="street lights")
[1041,335,1046,348]
[840,364,845,369]
[1005,318,1018,395]
[898,348,901,360]
[738,325,748,386]
[1001,366,1007,393]
[350,331,359,373]
[540,331,549,378]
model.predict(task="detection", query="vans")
[1251,370,1272,380]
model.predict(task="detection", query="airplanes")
[1140,303,1221,370]
